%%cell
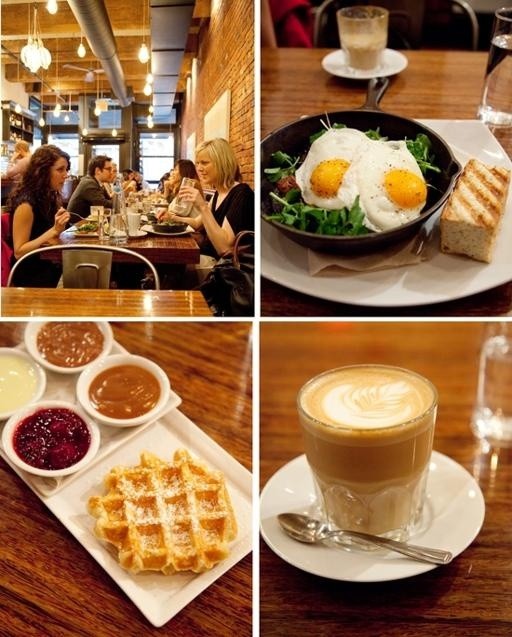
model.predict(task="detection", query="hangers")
[262,44,511,317]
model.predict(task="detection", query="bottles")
[112,177,124,215]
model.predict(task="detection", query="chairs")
[313,0,481,50]
[8,244,162,290]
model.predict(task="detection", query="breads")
[439,157,511,264]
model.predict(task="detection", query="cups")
[470,322,511,450]
[98,189,156,247]
[335,6,390,75]
[476,6,512,131]
[294,364,441,553]
[90,205,104,216]
[176,177,197,209]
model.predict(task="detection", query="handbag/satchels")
[199,227,254,318]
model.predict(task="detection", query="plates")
[73,232,98,237]
[140,225,195,236]
[261,118,512,308]
[260,450,487,584]
[320,48,409,80]
[150,203,169,207]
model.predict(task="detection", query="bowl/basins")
[24,321,114,375]
[3,401,101,478]
[75,353,170,428]
[0,347,47,422]
[261,78,465,258]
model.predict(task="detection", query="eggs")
[294,127,428,233]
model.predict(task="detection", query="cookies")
[87,450,238,577]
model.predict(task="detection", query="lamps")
[14,0,120,137]
[138,0,155,129]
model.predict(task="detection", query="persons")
[156,137,254,290]
[101,160,206,247]
[261,0,313,48]
[7,144,72,287]
[66,156,113,228]
[1,140,32,181]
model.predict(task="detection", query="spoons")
[276,514,453,566]
[63,210,98,222]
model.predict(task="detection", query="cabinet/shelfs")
[2,100,34,146]
